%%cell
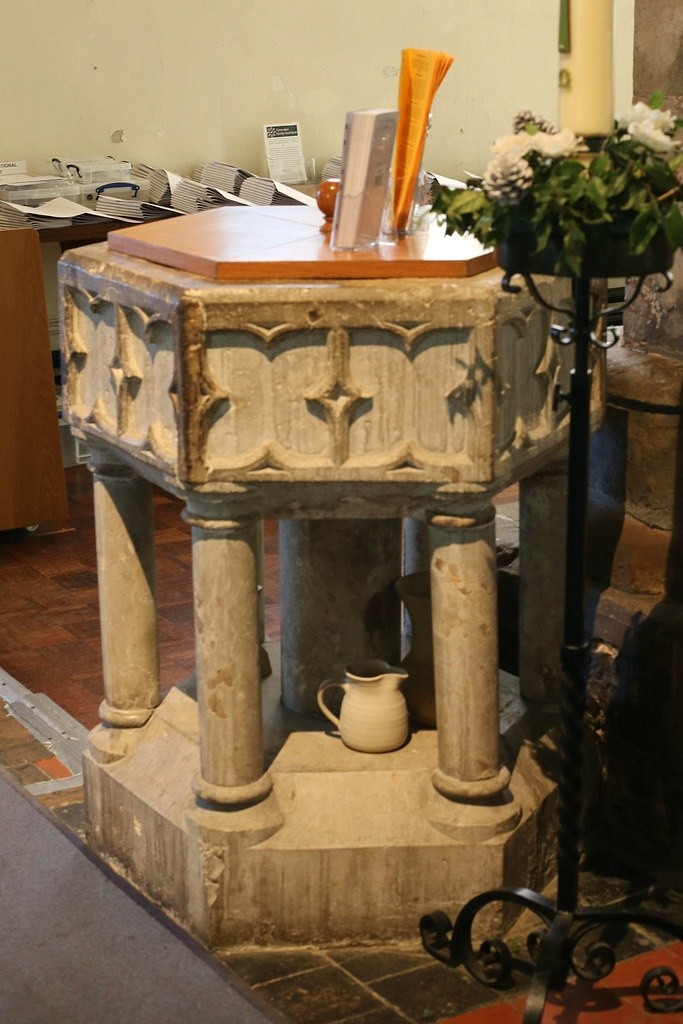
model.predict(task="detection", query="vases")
[494,214,674,275]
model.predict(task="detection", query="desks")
[56,198,606,807]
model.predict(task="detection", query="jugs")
[316,659,410,753]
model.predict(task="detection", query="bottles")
[395,571,436,727]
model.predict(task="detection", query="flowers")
[422,97,683,274]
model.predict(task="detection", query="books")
[329,104,400,249]
